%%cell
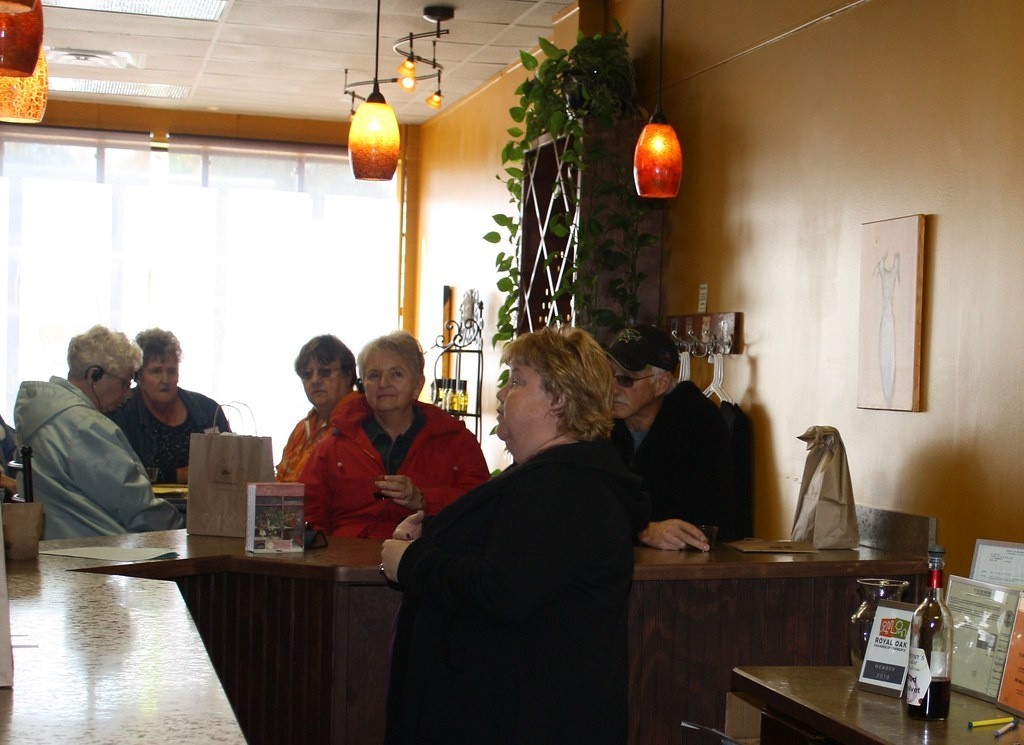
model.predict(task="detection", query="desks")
[725,668,1024,745]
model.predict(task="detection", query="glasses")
[299,365,346,382]
[103,371,132,391]
[611,368,665,387]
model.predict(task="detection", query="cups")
[369,475,391,500]
[144,467,159,485]
[685,525,720,552]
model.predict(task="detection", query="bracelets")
[417,491,424,511]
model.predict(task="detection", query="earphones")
[85,365,104,381]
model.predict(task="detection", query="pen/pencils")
[967,717,1016,728]
[995,719,1019,737]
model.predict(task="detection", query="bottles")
[907,545,953,721]
[432,379,468,414]
[850,579,911,682]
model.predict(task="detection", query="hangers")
[676,352,735,407]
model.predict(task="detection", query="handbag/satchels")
[186,401,274,538]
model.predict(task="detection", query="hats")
[600,325,678,373]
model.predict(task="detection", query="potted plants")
[488,25,650,390]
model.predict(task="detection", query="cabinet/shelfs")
[516,117,662,354]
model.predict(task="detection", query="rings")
[379,563,384,572]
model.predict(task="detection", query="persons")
[603,324,736,553]
[274,334,361,483]
[102,328,231,483]
[379,326,651,745]
[15,325,187,539]
[298,329,490,542]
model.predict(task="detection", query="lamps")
[348,0,401,181]
[0,0,47,124]
[344,6,458,118]
[634,0,683,199]
